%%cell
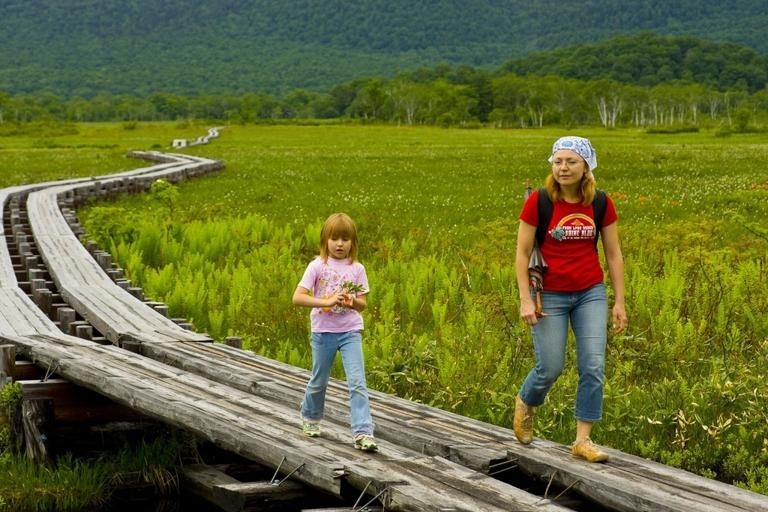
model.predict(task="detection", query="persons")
[292,212,379,453]
[512,135,627,464]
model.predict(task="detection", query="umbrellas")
[525,175,550,318]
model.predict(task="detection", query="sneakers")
[512,392,536,445]
[352,433,378,451]
[571,435,610,464]
[302,420,322,437]
